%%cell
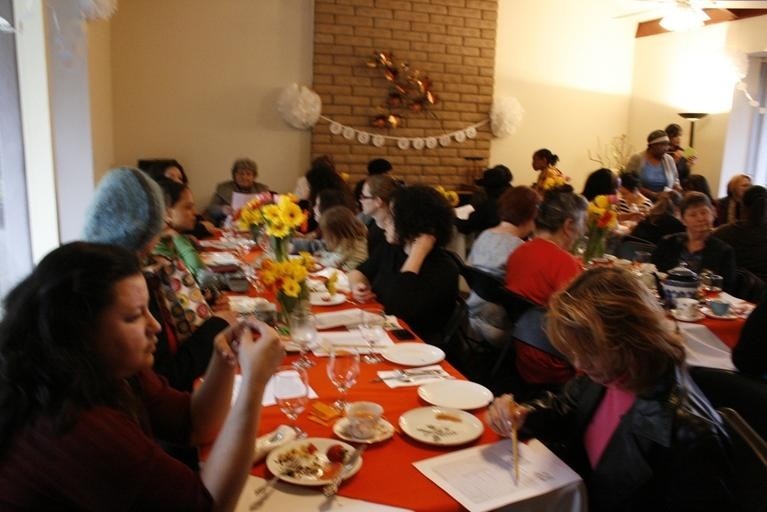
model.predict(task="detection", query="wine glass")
[276,311,359,431]
[356,307,387,366]
[703,274,723,302]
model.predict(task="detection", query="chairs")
[412,245,588,471]
[702,404,765,511]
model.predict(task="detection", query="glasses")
[358,192,377,200]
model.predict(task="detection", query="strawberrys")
[327,444,349,463]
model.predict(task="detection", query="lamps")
[657,6,708,35]
[675,108,708,152]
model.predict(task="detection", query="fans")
[605,0,767,29]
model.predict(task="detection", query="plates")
[669,311,704,323]
[704,311,737,322]
[396,406,484,446]
[585,249,655,277]
[204,236,251,264]
[292,245,349,308]
[380,342,449,367]
[414,379,496,410]
[267,439,360,486]
[330,416,395,443]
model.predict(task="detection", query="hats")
[83,167,167,249]
[647,129,673,146]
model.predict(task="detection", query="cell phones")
[392,329,415,340]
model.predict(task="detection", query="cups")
[343,398,383,436]
[676,297,698,315]
[712,300,730,316]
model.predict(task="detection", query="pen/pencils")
[507,392,519,487]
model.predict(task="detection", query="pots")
[650,261,703,304]
